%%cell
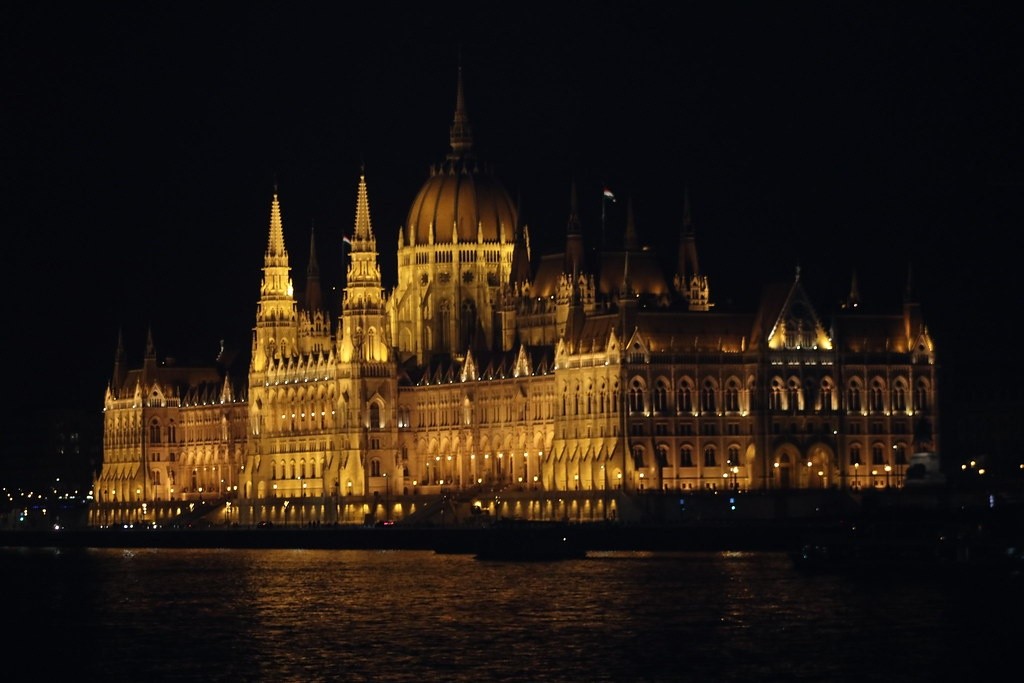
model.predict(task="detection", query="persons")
[307,519,321,529]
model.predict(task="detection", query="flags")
[342,232,352,246]
[600,182,618,209]
